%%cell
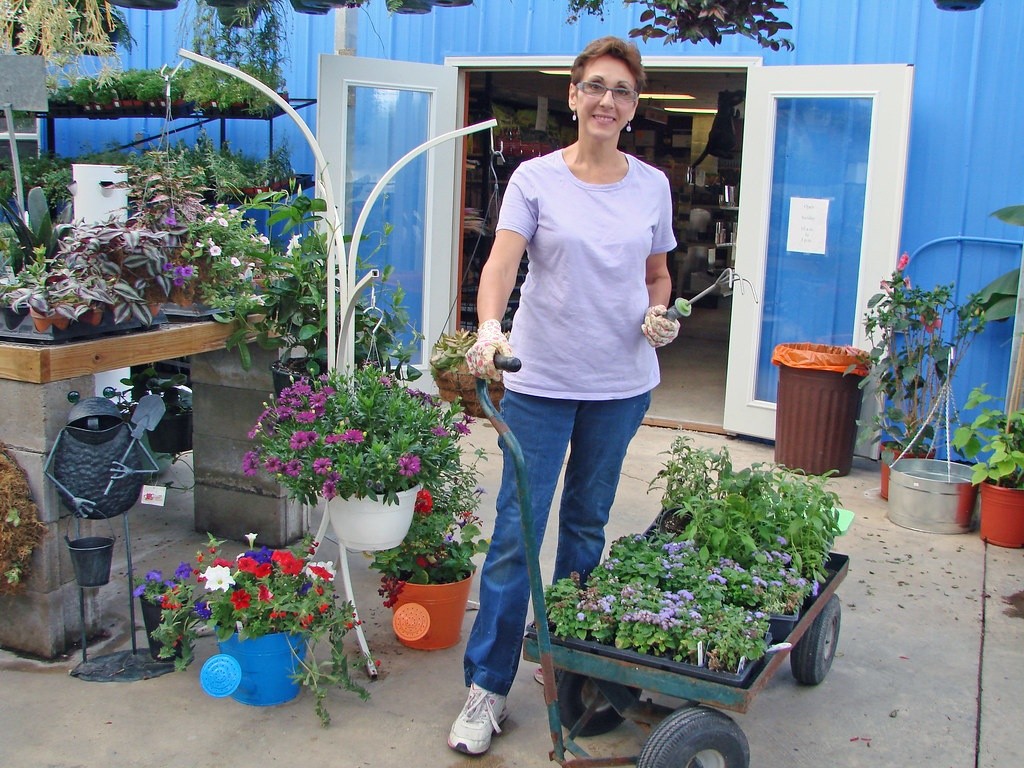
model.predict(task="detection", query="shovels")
[103,395,166,496]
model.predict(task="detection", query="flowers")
[240,363,477,498]
[192,527,379,729]
[842,257,985,460]
[185,196,304,313]
[118,560,211,673]
[367,490,489,607]
[540,529,822,666]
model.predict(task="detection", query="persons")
[448,37,681,756]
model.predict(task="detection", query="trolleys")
[476,355,851,768]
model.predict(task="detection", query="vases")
[138,581,189,662]
[878,439,936,501]
[523,604,773,687]
[200,624,311,706]
[326,482,425,552]
[743,603,800,643]
[383,564,474,651]
[887,346,979,535]
[0,0,474,53]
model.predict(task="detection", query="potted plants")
[223,195,427,397]
[949,385,1024,549]
[429,150,525,417]
[48,59,289,113]
[212,146,296,195]
[119,367,194,454]
[642,433,835,582]
[0,184,171,332]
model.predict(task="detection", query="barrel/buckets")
[888,458,979,533]
[65,506,117,587]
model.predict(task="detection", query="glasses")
[576,81,638,104]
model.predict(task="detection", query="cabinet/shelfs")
[682,201,739,310]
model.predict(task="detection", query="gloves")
[641,305,681,348]
[465,319,512,381]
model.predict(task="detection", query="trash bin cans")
[771,342,872,478]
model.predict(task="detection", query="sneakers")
[447,682,509,755]
[534,666,545,686]
